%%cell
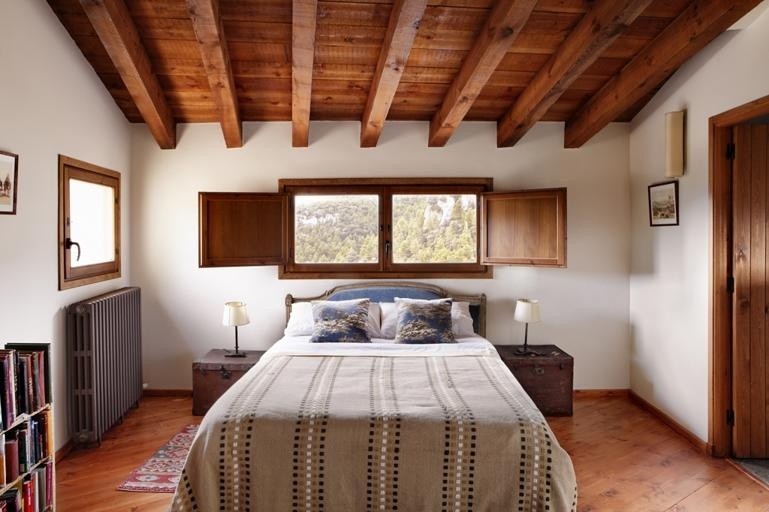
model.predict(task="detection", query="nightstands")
[494,344,574,416]
[192,349,266,413]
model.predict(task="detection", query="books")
[0,342,52,432]
[0,462,52,512]
[0,410,53,487]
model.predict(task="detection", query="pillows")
[392,296,457,345]
[283,302,380,338]
[308,299,372,343]
[380,301,475,340]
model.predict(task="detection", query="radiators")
[66,285,143,451]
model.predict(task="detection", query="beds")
[171,281,578,512]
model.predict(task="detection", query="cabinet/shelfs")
[0,342,56,512]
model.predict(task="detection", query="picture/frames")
[648,180,680,227]
[0,150,20,216]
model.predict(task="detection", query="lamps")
[514,298,542,356]
[663,111,685,179]
[222,301,250,358]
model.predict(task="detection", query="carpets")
[118,421,201,494]
